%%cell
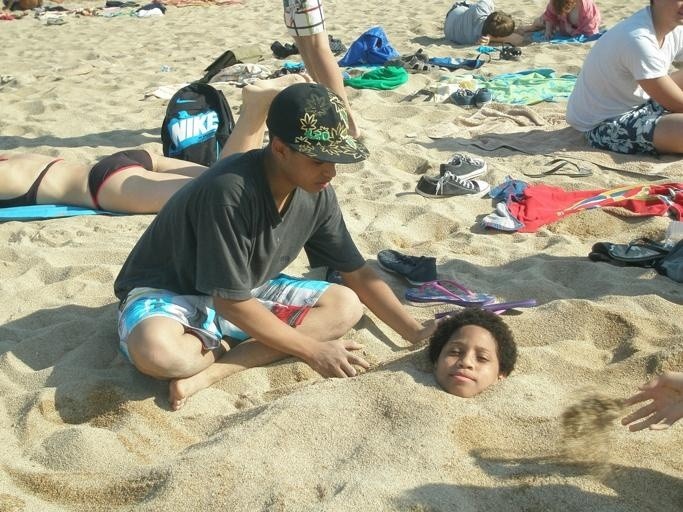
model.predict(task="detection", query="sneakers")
[379,246,437,288]
[416,172,491,200]
[475,86,494,109]
[449,89,478,110]
[439,153,489,178]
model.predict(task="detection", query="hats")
[268,83,373,164]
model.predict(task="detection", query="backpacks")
[160,83,236,164]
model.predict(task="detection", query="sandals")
[385,48,442,76]
[497,39,523,62]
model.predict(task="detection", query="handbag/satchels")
[338,26,399,66]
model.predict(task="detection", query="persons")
[429,309,516,397]
[622,370,683,432]
[0,72,316,214]
[519,0,598,39]
[285,0,360,140]
[565,0,683,154]
[113,83,451,411]
[444,0,524,46]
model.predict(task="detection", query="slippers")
[608,235,671,264]
[522,157,594,178]
[404,279,536,319]
